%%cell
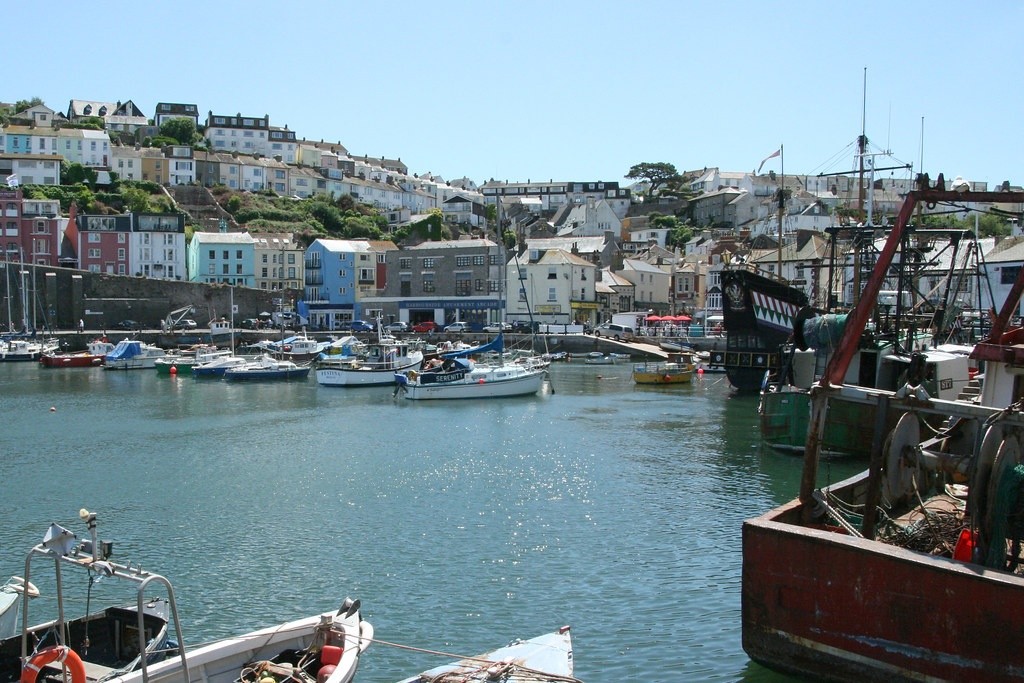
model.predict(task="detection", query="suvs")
[351,321,373,333]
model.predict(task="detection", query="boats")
[585,351,618,364]
[38,338,115,367]
[105,334,166,369]
[554,352,570,360]
[0,507,574,683]
[250,333,480,388]
[154,337,233,373]
[632,357,694,385]
[720,59,1024,683]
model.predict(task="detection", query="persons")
[160,318,165,329]
[255,318,273,330]
[79,316,84,332]
[512,320,518,328]
[11,321,16,331]
[571,317,593,334]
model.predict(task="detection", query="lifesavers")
[20,645,86,683]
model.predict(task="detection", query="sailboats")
[390,188,551,400]
[225,278,310,379]
[190,285,251,376]
[0,237,60,362]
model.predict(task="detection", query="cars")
[113,320,136,329]
[384,322,409,332]
[410,321,541,333]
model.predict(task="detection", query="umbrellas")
[645,315,692,330]
[259,310,271,316]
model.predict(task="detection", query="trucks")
[604,312,647,336]
[176,319,197,329]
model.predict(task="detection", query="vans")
[595,324,633,343]
